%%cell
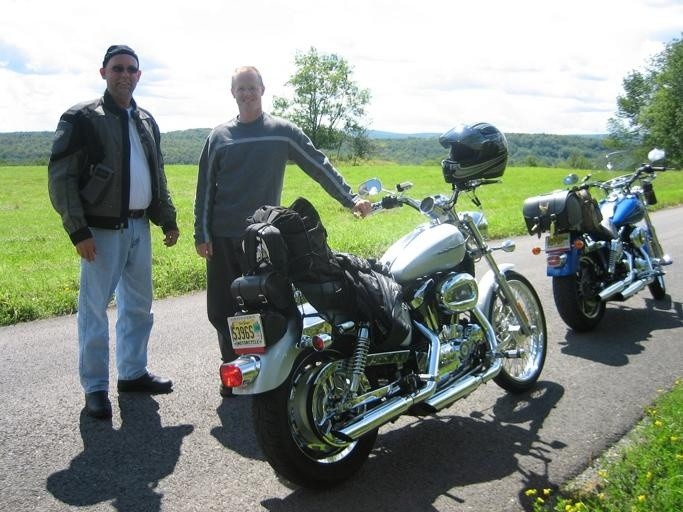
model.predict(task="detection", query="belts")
[127,207,151,220]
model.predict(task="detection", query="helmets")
[439,120,510,184]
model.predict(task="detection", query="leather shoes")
[117,372,173,394]
[84,389,113,418]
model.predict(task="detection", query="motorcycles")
[520,147,673,334]
[220,124,548,490]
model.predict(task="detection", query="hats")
[102,44,140,68]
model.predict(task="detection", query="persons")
[46,44,180,419]
[193,67,371,398]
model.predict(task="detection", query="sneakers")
[219,383,238,399]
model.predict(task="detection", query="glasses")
[104,64,139,74]
